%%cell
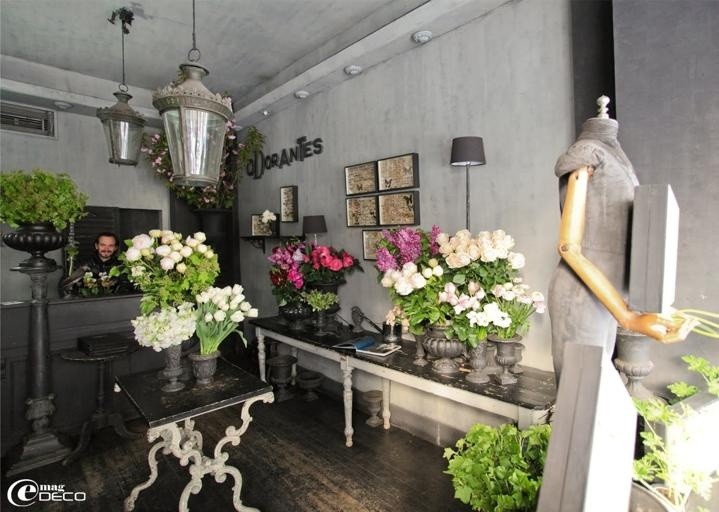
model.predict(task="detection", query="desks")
[248,314,557,447]
[115,356,275,512]
[61,343,143,439]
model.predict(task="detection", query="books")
[77,332,130,357]
[332,335,402,358]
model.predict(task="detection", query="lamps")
[96,6,145,165]
[303,215,327,245]
[153,1,235,187]
[450,137,485,231]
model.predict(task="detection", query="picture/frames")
[251,212,279,234]
[280,185,298,223]
[344,153,420,261]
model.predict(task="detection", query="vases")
[162,345,220,393]
[192,212,228,237]
[285,282,346,335]
[413,322,524,384]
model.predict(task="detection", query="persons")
[546,116,700,390]
[77,232,136,292]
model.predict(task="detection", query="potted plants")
[0,170,89,477]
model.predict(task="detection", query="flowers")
[269,240,365,310]
[142,124,265,208]
[109,230,259,353]
[375,225,546,346]
